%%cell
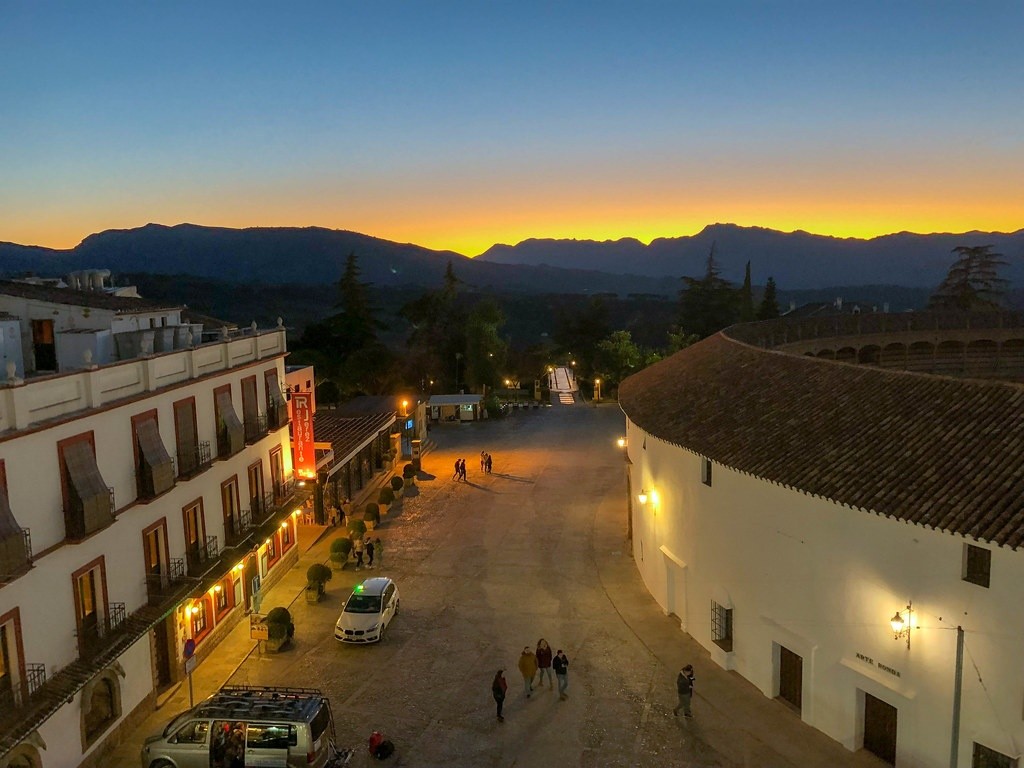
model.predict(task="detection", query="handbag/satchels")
[376,741,395,760]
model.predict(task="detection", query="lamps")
[618,436,628,448]
[890,600,912,650]
[638,489,657,516]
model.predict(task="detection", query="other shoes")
[560,693,567,701]
[672,707,680,718]
[531,689,533,691]
[684,711,692,719]
[527,695,530,698]
[496,714,504,722]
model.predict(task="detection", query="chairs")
[296,500,315,526]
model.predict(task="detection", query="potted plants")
[259,606,295,652]
[305,563,332,602]
[328,458,421,570]
[380,447,397,469]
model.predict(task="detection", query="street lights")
[596,378,600,403]
[505,379,510,403]
[548,368,552,389]
[572,360,576,381]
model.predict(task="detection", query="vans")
[140,686,356,768]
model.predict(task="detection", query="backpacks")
[369,731,381,754]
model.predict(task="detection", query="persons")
[536,638,554,689]
[452,459,466,481]
[492,670,507,722]
[553,650,570,700]
[224,722,244,768]
[355,537,383,571]
[672,665,695,718]
[330,499,351,526]
[480,451,492,474]
[519,646,538,697]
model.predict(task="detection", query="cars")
[335,578,400,642]
